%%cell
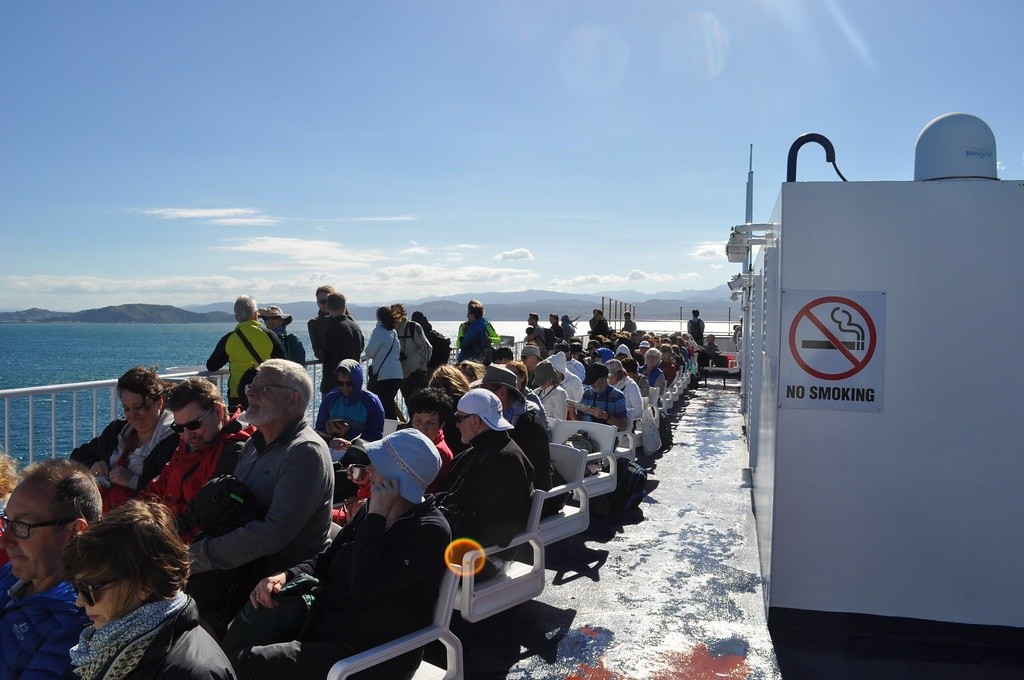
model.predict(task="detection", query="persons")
[733,318,742,352]
[0,286,699,680]
[698,335,717,367]
[687,309,705,347]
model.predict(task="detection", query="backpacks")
[613,457,648,513]
[562,429,599,477]
[538,327,556,351]
[428,330,452,370]
[179,473,266,611]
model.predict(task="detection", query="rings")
[256,592,260,594]
[95,472,98,474]
[340,444,343,446]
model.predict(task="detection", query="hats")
[457,388,515,432]
[259,305,293,326]
[531,360,556,385]
[520,345,543,361]
[638,341,650,348]
[468,364,526,403]
[581,362,608,384]
[364,428,442,506]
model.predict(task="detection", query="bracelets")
[603,413,611,421]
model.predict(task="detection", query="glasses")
[586,347,594,351]
[170,404,216,433]
[71,574,123,605]
[244,381,299,397]
[454,412,473,424]
[0,512,71,540]
[519,355,530,360]
[334,379,353,387]
[319,299,327,305]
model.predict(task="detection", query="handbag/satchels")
[546,467,572,508]
[367,365,379,391]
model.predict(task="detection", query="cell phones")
[333,419,345,428]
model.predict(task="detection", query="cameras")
[399,352,406,360]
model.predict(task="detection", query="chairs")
[326,337,745,680]
